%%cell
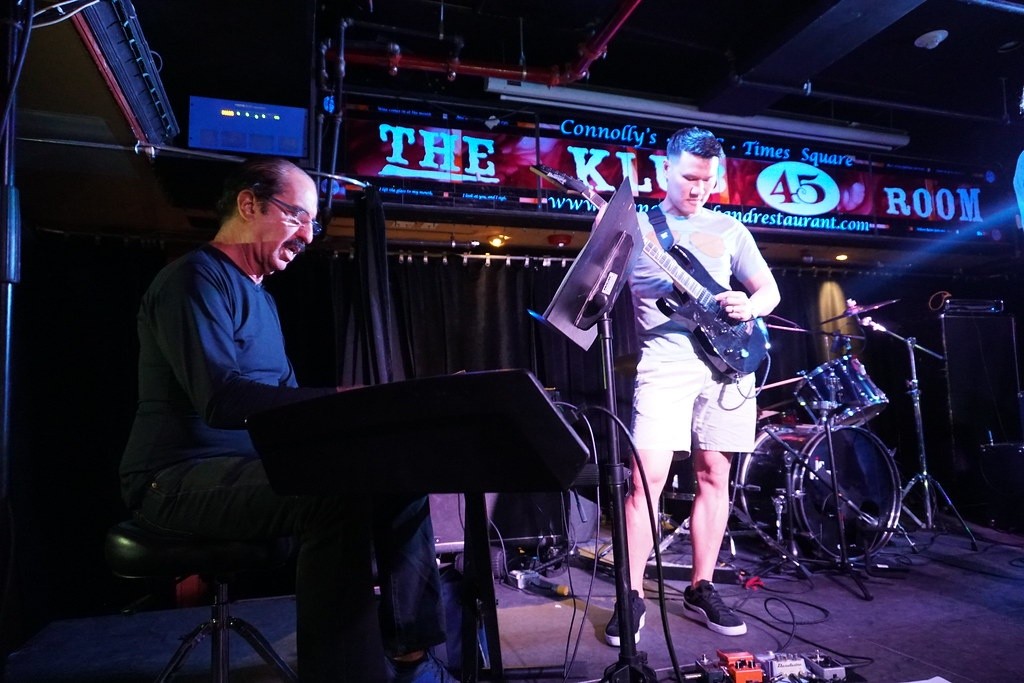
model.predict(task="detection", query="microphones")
[858,513,879,532]
[830,335,845,353]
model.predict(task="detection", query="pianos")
[240,365,592,505]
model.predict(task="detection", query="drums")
[734,423,904,568]
[792,353,889,429]
[660,487,696,536]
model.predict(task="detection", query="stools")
[117,519,299,683]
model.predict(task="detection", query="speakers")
[979,443,1024,534]
[938,314,1024,518]
[428,491,570,554]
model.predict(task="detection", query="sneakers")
[605,590,647,647]
[683,580,748,636]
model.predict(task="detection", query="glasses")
[254,190,323,236]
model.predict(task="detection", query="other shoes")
[385,652,458,683]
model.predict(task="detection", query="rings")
[731,305,735,313]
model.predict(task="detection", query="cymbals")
[818,298,902,326]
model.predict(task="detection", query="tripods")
[749,315,979,603]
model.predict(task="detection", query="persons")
[591,128,782,646]
[120,156,467,683]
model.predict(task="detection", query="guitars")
[529,162,770,381]
